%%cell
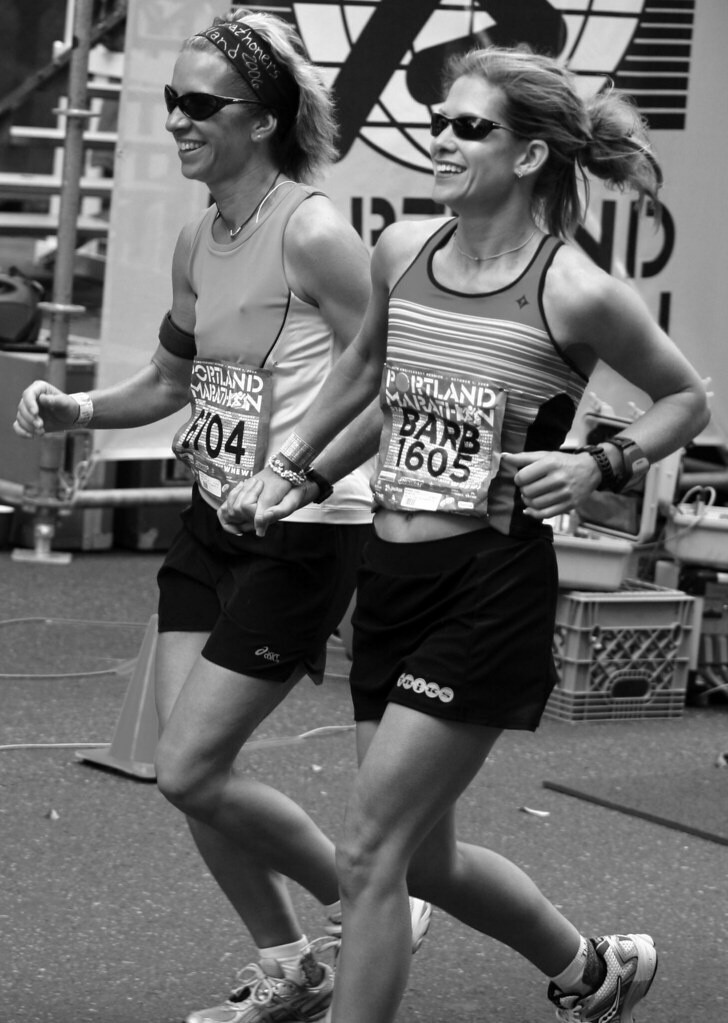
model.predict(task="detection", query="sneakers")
[185,961,334,1023]
[548,934,658,1023]
[301,896,433,1010]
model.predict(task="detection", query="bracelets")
[67,391,94,430]
[304,466,334,505]
[266,455,303,486]
[277,430,318,470]
[575,447,614,491]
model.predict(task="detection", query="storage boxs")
[542,578,697,729]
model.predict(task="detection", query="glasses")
[164,84,265,122]
[430,112,535,142]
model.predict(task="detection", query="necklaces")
[452,229,539,262]
[216,170,282,240]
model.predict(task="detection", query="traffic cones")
[75,613,159,781]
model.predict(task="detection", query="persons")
[13,7,432,1023]
[216,47,713,1022]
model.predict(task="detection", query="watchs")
[606,436,650,494]
